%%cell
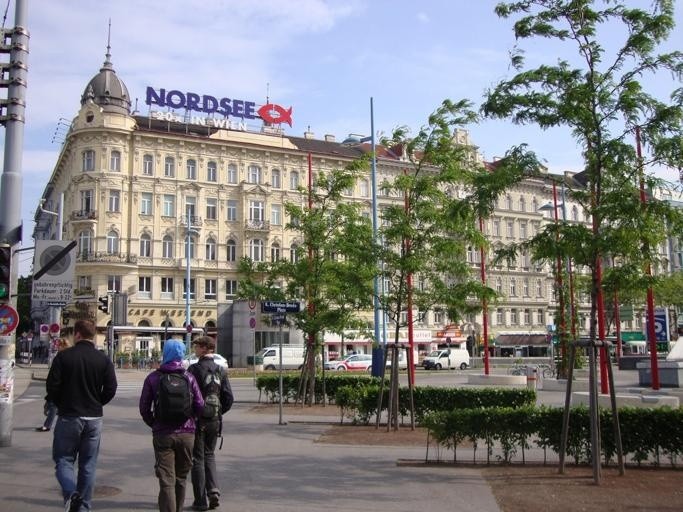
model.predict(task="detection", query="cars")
[324,353,374,372]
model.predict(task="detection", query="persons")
[138,338,206,512]
[44,318,119,511]
[186,335,233,510]
[36,337,71,431]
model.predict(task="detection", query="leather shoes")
[36,426,50,432]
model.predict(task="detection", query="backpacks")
[196,362,224,449]
[156,369,194,425]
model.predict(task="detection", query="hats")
[193,335,216,350]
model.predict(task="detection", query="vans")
[252,343,306,371]
[421,348,470,370]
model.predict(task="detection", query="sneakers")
[65,491,84,511]
[208,493,220,509]
[190,503,207,511]
